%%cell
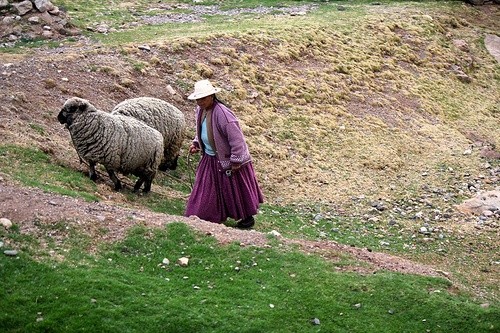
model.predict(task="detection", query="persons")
[225,228,226,232]
[183,79,264,228]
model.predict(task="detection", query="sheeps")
[109,96,186,171]
[57,97,165,195]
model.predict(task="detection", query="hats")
[187,79,222,100]
